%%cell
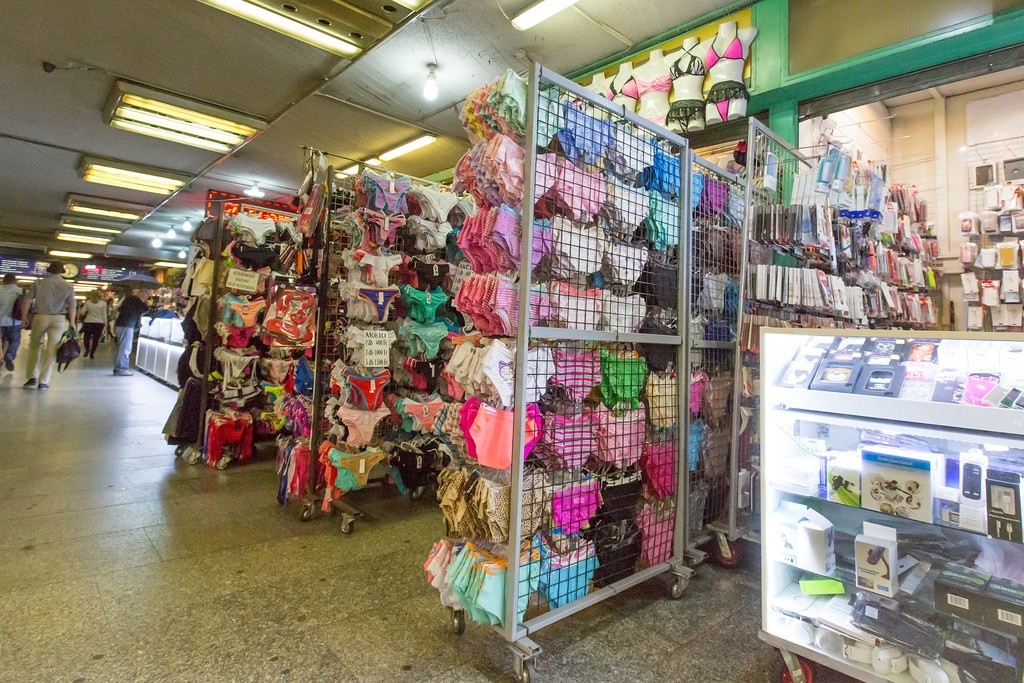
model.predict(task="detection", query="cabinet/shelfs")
[754,328,1024,683]
[134,315,187,388]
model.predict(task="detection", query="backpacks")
[13,287,33,320]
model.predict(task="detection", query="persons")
[77,290,119,360]
[113,286,150,375]
[0,272,33,372]
[22,261,77,391]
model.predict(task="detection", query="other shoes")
[38,383,48,389]
[4,356,15,372]
[24,378,37,387]
[114,369,132,376]
[85,349,89,358]
[90,355,95,359]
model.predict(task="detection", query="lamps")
[64,192,152,221]
[176,246,187,258]
[151,234,162,248]
[52,229,116,247]
[182,217,192,231]
[166,225,176,239]
[97,80,266,155]
[44,246,97,260]
[76,153,195,197]
[423,63,439,102]
[243,179,265,198]
[58,215,132,234]
[376,132,436,163]
[334,156,382,181]
[509,0,580,32]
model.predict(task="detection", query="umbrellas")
[111,272,162,290]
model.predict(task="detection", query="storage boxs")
[795,508,836,575]
[781,451,820,497]
[772,500,807,565]
[824,453,862,508]
[984,576,1024,639]
[932,563,991,626]
[852,534,899,598]
[777,335,942,398]
[861,444,944,526]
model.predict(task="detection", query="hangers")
[206,77,744,575]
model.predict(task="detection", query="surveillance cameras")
[43,62,55,73]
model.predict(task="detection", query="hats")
[47,262,65,273]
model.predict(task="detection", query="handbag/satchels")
[56,334,81,373]
